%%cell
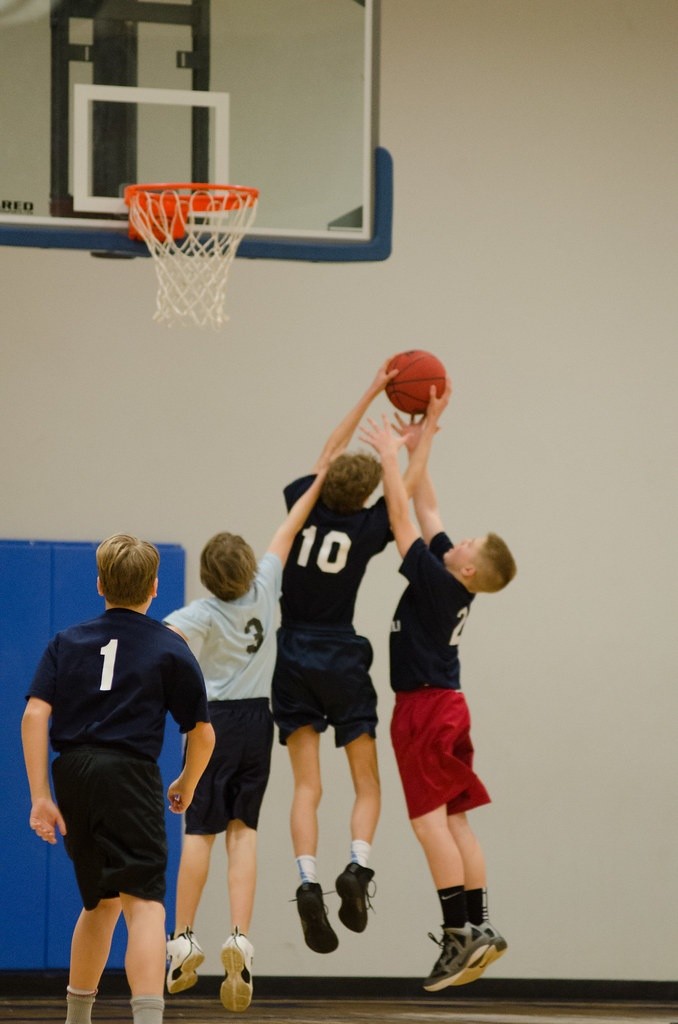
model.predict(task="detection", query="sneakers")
[167,926,203,994]
[335,863,376,933]
[422,921,493,993]
[289,883,338,954]
[220,926,254,1013]
[449,922,507,986]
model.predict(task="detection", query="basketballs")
[384,350,448,415]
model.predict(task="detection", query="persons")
[359,411,517,991]
[161,462,328,1012]
[269,351,453,954]
[21,533,215,1024]
